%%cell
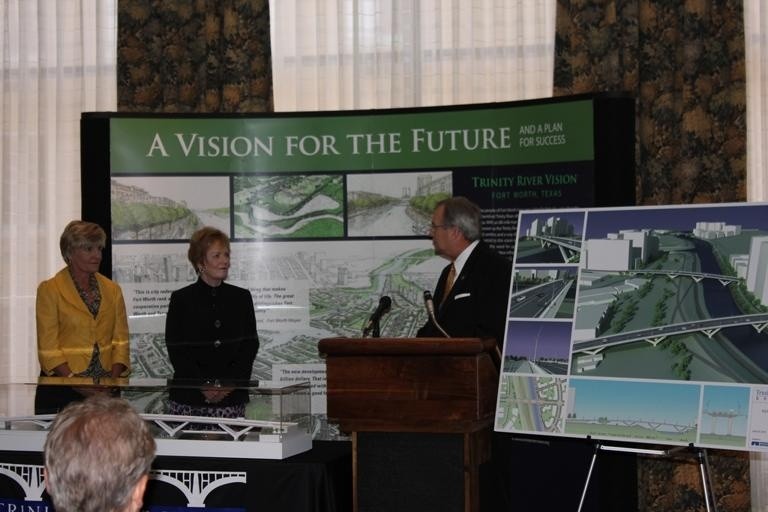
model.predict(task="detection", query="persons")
[42,396,156,511]
[165,228,260,434]
[36,220,129,416]
[416,196,513,361]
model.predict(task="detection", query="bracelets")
[68,372,76,378]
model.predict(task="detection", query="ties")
[439,263,456,308]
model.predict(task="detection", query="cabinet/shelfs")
[0,373,318,511]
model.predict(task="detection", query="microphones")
[423,289,435,318]
[370,296,391,325]
